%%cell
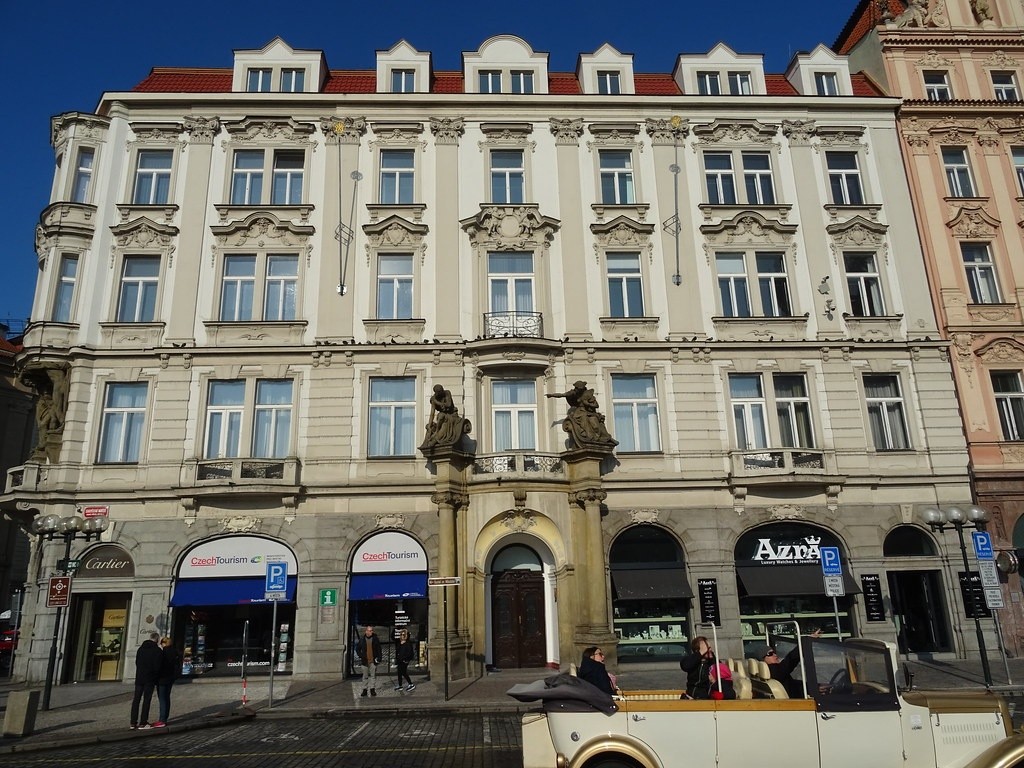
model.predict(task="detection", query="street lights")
[31,513,109,712]
[921,506,994,691]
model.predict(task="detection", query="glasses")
[400,634,405,636]
[766,650,776,656]
[593,652,603,657]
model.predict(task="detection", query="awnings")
[347,572,428,601]
[736,564,864,598]
[167,576,297,607]
[611,568,696,602]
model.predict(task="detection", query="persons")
[744,629,823,699]
[577,646,620,701]
[543,381,618,445]
[394,628,415,693]
[419,384,462,448]
[357,624,382,697]
[680,637,737,700]
[130,633,177,730]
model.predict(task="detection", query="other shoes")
[130,725,135,729]
[395,687,403,691]
[361,690,367,696]
[152,722,166,728]
[137,724,153,729]
[406,684,416,692]
[370,688,376,695]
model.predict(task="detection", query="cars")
[518,617,1024,768]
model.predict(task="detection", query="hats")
[709,664,732,680]
[753,644,772,661]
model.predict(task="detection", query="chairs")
[726,658,789,701]
